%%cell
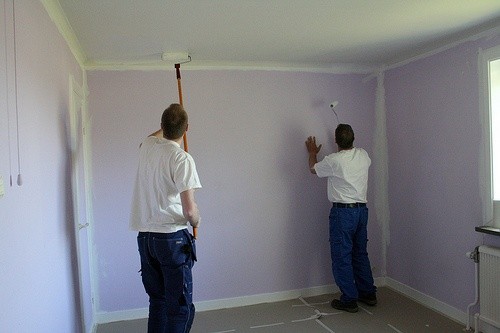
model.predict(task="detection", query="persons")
[303,123,378,313]
[130,103,203,333]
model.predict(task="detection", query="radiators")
[462,245,500,333]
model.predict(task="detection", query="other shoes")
[357,292,377,305]
[332,299,358,313]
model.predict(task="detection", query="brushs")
[329,100,340,124]
[161,53,197,240]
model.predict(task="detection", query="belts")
[333,202,366,208]
